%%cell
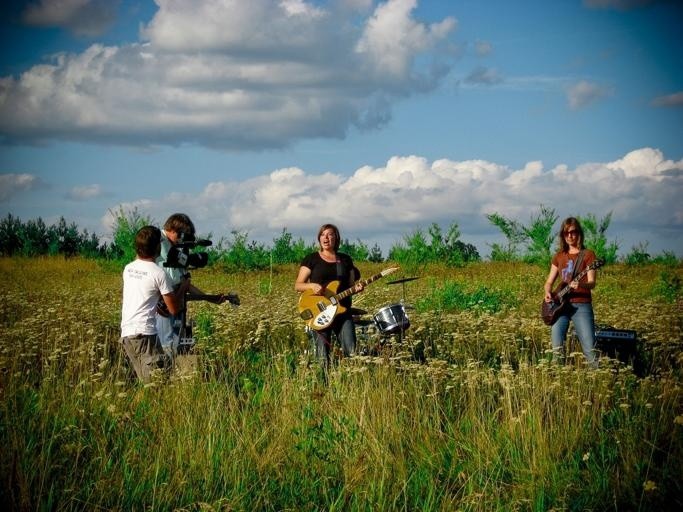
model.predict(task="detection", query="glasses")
[563,230,578,237]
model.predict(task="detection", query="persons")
[116,224,192,385]
[154,213,226,359]
[293,222,365,386]
[543,216,600,368]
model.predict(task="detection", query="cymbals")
[349,307,368,315]
[386,277,418,287]
[297,266,399,333]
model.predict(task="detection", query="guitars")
[542,257,606,326]
[186,292,240,305]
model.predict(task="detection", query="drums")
[373,304,409,333]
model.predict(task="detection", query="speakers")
[589,330,636,369]
